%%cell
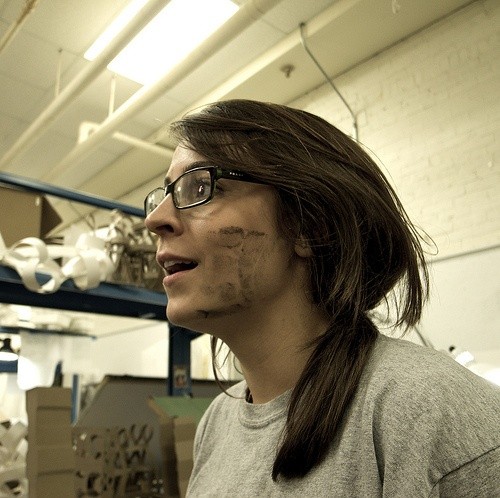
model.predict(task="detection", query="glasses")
[144,165,270,218]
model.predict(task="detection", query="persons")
[144,100,500,498]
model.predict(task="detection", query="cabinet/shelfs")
[0,171,189,497]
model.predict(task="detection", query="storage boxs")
[1,182,63,246]
[75,372,240,497]
